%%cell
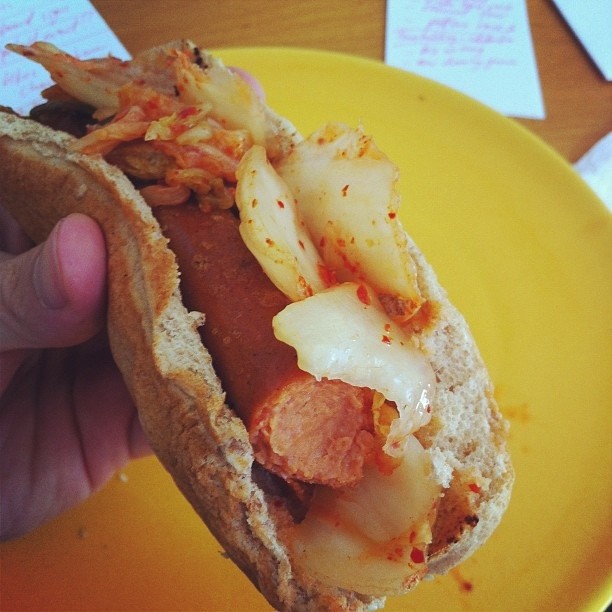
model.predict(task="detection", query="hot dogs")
[0,38,510,611]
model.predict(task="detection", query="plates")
[1,47,612,612]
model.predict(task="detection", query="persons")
[0,213,155,545]
